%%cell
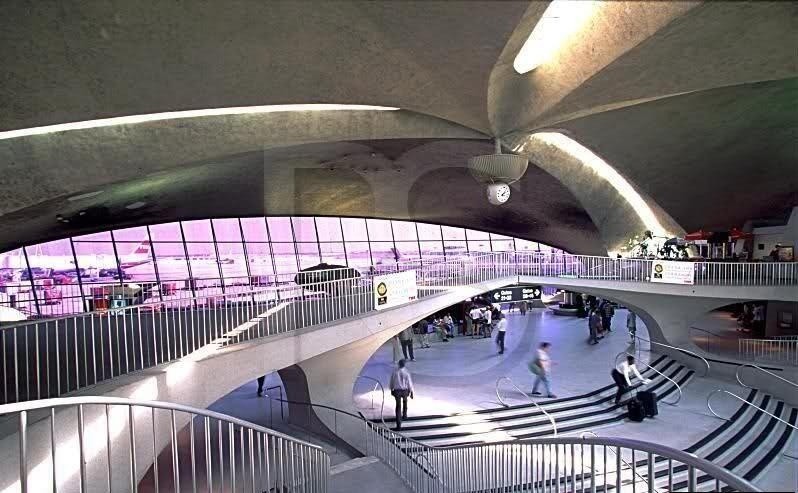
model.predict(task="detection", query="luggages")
[636,383,657,417]
[626,386,645,421]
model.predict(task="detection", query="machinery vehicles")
[43,289,63,306]
[217,258,234,264]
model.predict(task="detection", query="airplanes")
[0,232,157,291]
[386,247,493,269]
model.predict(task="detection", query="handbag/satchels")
[527,360,543,377]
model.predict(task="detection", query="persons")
[633,230,657,255]
[257,375,266,397]
[394,293,636,362]
[734,302,766,330]
[389,358,414,430]
[530,341,557,397]
[610,354,646,404]
